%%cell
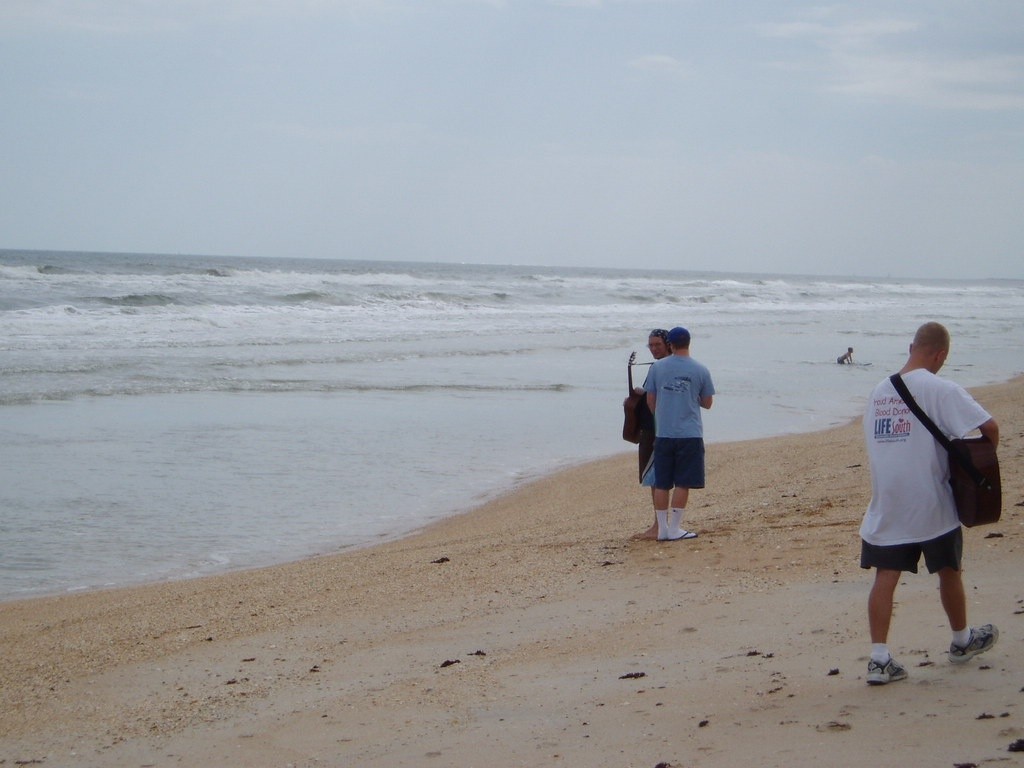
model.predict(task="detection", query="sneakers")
[865,652,908,684]
[949,623,999,664]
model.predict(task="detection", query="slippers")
[656,530,698,541]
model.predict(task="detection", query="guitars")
[948,435,1002,528]
[622,351,647,445]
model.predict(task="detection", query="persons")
[859,321,1000,685]
[623,327,715,541]
[837,347,854,365]
